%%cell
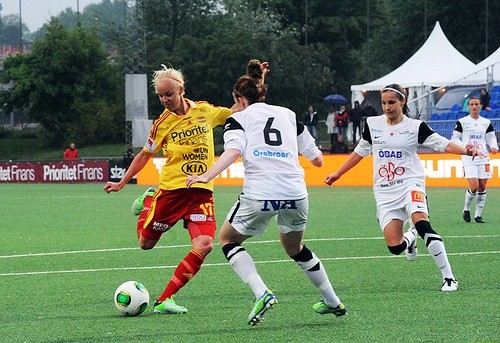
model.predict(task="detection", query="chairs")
[429,85,500,139]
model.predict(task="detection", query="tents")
[350,22,500,142]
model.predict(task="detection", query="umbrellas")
[323,94,348,104]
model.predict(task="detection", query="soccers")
[113,281,150,316]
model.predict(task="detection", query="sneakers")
[153,294,188,314]
[475,216,485,223]
[132,187,156,216]
[404,228,418,260]
[441,278,459,292]
[463,211,471,222]
[313,299,347,318]
[248,290,278,325]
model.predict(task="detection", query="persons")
[64,142,78,160]
[186,59,347,326]
[479,89,491,110]
[324,84,487,292]
[304,104,323,148]
[325,101,378,154]
[103,62,270,314]
[450,96,499,223]
